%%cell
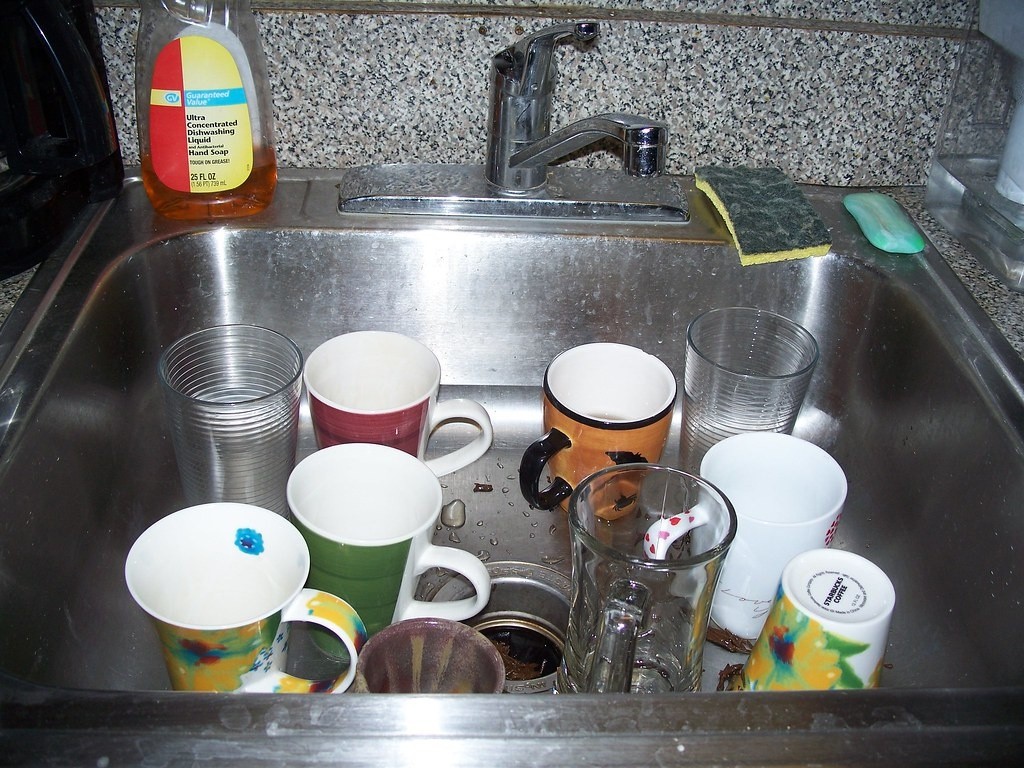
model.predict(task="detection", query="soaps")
[844,191,926,255]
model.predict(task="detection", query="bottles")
[135,0,277,219]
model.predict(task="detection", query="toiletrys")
[131,1,278,222]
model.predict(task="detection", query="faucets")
[336,22,691,223]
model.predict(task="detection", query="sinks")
[1,227,1023,694]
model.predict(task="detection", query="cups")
[157,323,303,523]
[355,617,506,694]
[519,343,678,520]
[677,307,820,472]
[286,443,490,645]
[124,502,368,693]
[302,330,493,478]
[551,463,738,695]
[729,548,896,692]
[700,433,848,639]
